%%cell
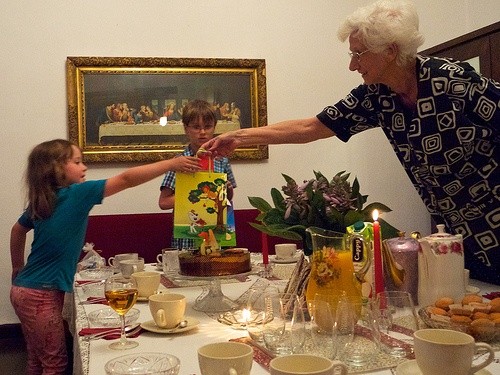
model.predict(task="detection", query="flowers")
[248,168,400,250]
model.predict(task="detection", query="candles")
[372,208,387,308]
[261,232,270,263]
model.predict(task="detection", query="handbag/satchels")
[172,148,228,226]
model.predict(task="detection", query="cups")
[413,329,495,375]
[269,354,348,375]
[198,342,254,375]
[275,244,297,259]
[132,272,160,298]
[375,291,418,358]
[108,253,144,279]
[148,293,186,329]
[332,296,377,366]
[157,247,182,278]
[246,287,361,359]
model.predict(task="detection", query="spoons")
[170,320,187,332]
[81,323,140,339]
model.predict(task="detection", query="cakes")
[179,247,251,276]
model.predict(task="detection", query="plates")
[268,254,303,263]
[396,359,493,375]
[140,316,200,333]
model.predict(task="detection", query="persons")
[9,139,202,375]
[198,1,500,286]
[159,101,237,251]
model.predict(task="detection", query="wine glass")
[104,276,139,350]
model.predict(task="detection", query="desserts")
[427,294,500,342]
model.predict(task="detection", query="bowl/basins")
[419,303,500,342]
[271,262,297,280]
[79,267,181,375]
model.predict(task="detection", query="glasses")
[348,49,369,60]
[188,124,214,133]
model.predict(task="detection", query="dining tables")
[61,253,500,375]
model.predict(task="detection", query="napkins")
[75,278,101,286]
[78,323,142,341]
[86,295,109,307]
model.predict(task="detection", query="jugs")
[306,226,372,335]
[381,225,465,308]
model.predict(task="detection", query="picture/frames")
[62,54,272,162]
